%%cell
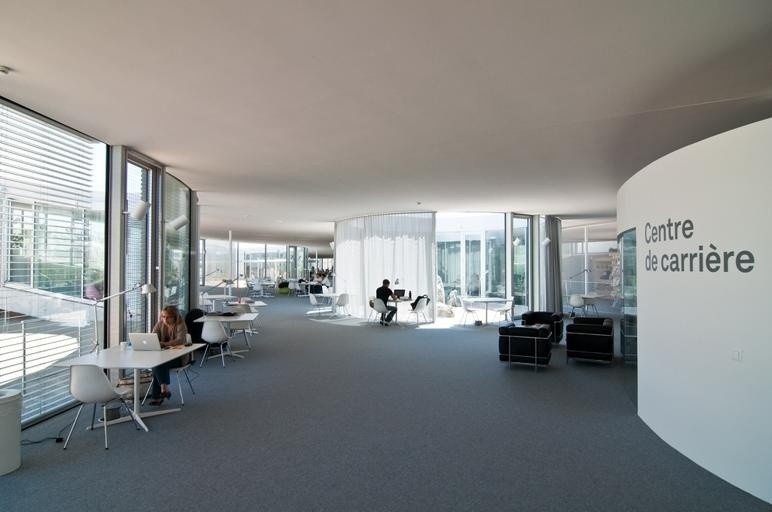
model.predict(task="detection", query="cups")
[120,341,128,353]
[237,298,241,303]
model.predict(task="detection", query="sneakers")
[160,391,171,399]
[148,398,163,406]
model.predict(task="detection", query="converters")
[56,438,63,442]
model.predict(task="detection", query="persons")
[376,279,397,327]
[149,306,187,406]
[309,266,331,288]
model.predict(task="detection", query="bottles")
[409,290,412,299]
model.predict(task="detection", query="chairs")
[461,288,636,374]
[194,266,353,321]
[52,321,261,457]
[365,291,432,328]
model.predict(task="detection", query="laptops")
[394,290,405,298]
[129,333,164,351]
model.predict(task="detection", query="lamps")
[539,236,552,248]
[120,197,151,222]
[161,212,190,231]
[90,283,158,355]
[512,234,523,249]
